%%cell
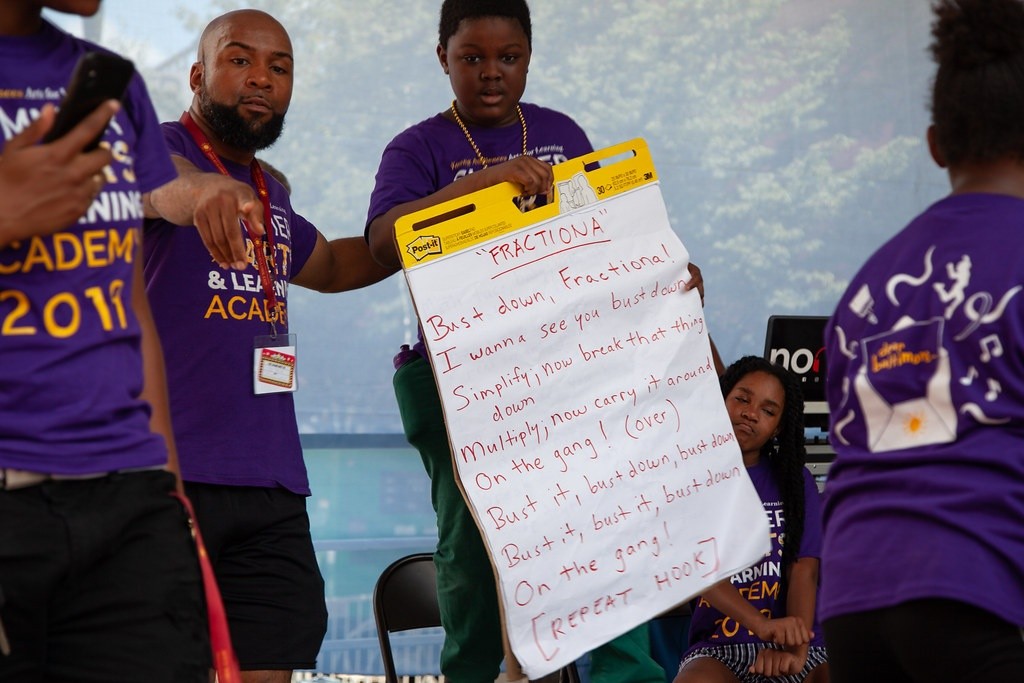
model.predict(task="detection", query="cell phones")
[41,52,135,153]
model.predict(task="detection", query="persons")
[142,8,403,683]
[364,0,704,683]
[819,0,1024,683]
[0,0,209,683]
[670,356,829,683]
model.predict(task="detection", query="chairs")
[372,552,441,683]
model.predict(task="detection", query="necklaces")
[450,98,527,212]
